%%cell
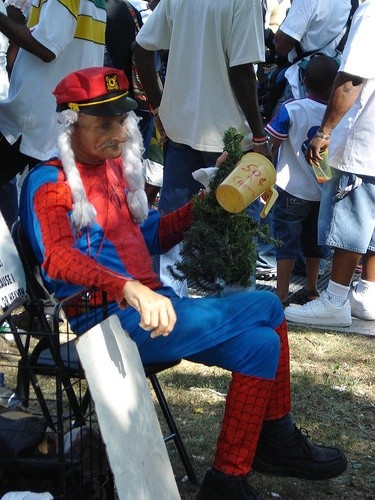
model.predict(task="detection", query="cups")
[216,152,277,213]
[310,149,333,184]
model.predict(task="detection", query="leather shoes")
[254,425,347,480]
[195,468,259,500]
[8,311,43,337]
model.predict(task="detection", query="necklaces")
[84,161,111,261]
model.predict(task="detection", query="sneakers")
[283,290,351,327]
[288,286,320,306]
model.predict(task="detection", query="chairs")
[0,163,199,500]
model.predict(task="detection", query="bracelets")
[316,130,331,140]
[153,106,159,116]
[252,137,268,146]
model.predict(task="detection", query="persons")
[0,0,375,327]
[20,67,348,500]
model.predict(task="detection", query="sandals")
[347,285,375,320]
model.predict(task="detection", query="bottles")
[0,322,27,347]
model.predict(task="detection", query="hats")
[52,67,138,117]
